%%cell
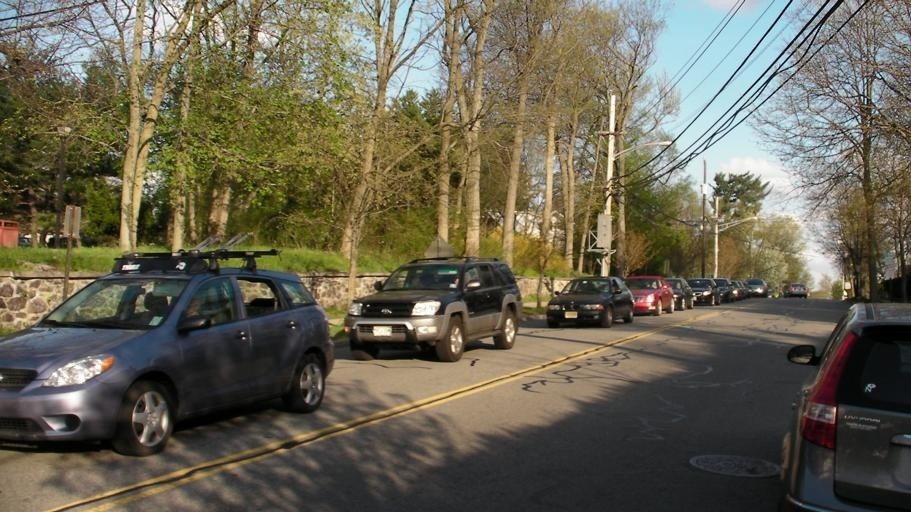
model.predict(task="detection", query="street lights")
[712,195,768,279]
[598,93,674,278]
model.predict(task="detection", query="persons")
[185,293,217,325]
[452,273,460,285]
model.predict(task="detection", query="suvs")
[342,253,524,364]
[0,249,337,456]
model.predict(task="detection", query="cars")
[782,283,808,299]
[543,275,637,328]
[713,277,770,304]
[775,300,911,512]
[621,276,676,317]
[686,278,721,306]
[664,278,695,311]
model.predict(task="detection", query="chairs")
[134,291,275,328]
